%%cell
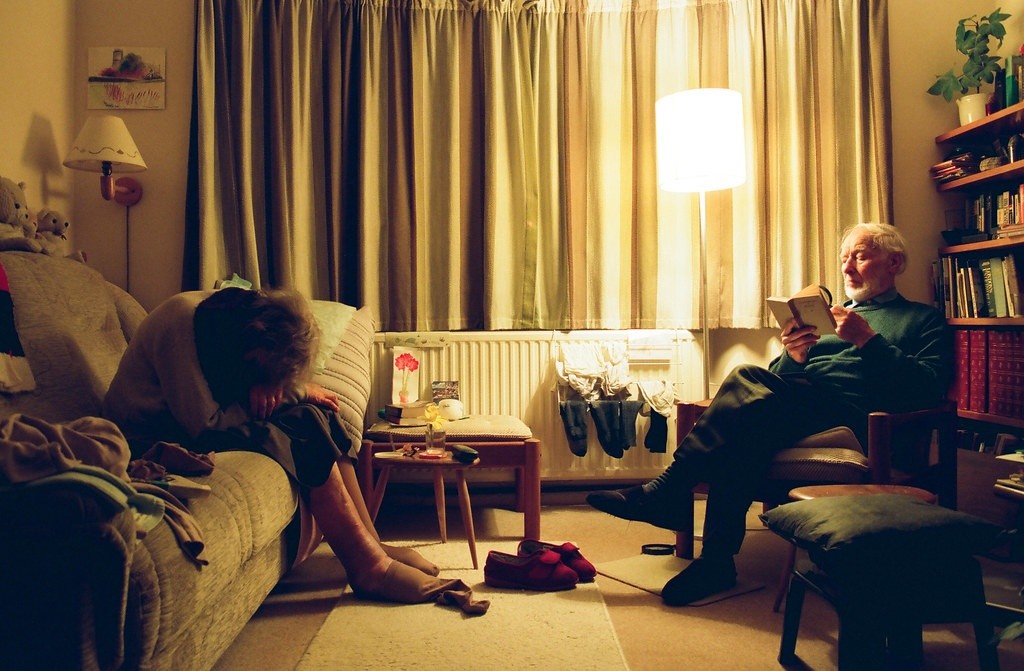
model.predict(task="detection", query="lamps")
[654,87,751,325]
[62,113,148,207]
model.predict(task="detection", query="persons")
[105,286,441,602]
[584,222,945,611]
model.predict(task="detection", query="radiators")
[370,329,705,485]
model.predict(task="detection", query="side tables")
[365,415,542,546]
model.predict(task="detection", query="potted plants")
[927,7,1011,126]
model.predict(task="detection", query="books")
[955,330,1023,417]
[962,183,1024,243]
[958,428,1015,456]
[765,283,838,337]
[930,253,1024,317]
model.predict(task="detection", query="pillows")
[758,494,1002,587]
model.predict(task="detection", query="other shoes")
[483,548,578,592]
[586,482,686,532]
[660,557,737,604]
[516,540,597,581]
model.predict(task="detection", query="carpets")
[592,528,811,606]
[294,540,629,671]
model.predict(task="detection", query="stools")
[367,450,482,569]
[776,539,995,671]
[773,485,937,612]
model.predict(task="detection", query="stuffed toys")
[0,176,87,264]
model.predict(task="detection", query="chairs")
[675,399,957,559]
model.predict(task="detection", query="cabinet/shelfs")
[932,97,1024,428]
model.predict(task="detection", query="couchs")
[0,251,377,670]
